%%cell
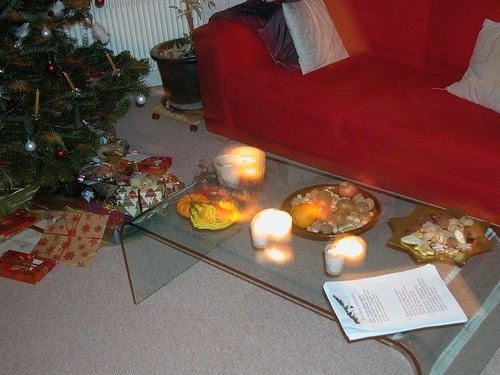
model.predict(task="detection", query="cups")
[213,154,241,190]
[250,221,270,248]
[324,245,344,276]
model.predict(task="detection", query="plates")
[281,184,382,242]
[386,203,497,265]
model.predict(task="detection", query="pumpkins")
[177,192,208,217]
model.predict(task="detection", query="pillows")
[445,19,500,114]
[281,0,350,75]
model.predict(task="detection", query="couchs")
[194,0,500,227]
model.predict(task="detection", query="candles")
[249,208,293,248]
[335,236,368,265]
[325,244,344,278]
[231,146,266,181]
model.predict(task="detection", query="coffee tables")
[120,158,500,375]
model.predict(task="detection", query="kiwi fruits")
[317,190,375,234]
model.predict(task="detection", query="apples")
[338,182,358,198]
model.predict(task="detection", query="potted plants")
[150,0,215,110]
